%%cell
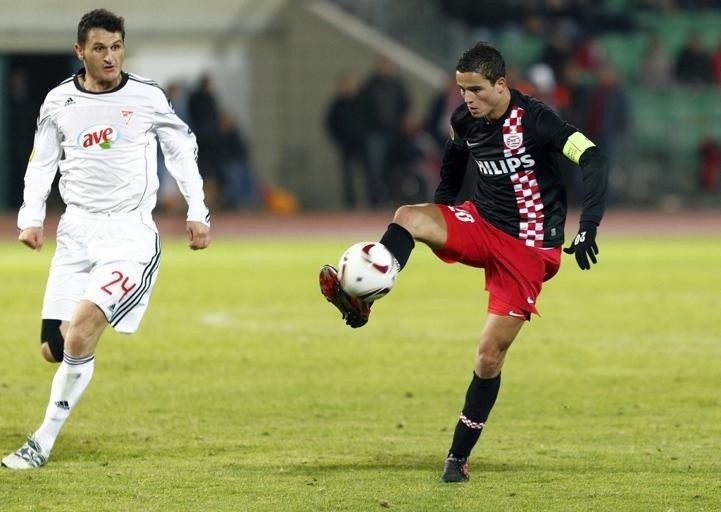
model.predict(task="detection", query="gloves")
[563,223,598,270]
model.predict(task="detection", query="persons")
[355,54,410,207]
[322,69,377,207]
[154,78,192,221]
[417,0,721,205]
[185,74,238,213]
[0,4,214,476]
[316,38,609,487]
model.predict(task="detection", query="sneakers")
[318,263,370,328]
[443,459,469,482]
[1,436,47,470]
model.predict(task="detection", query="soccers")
[338,241,398,304]
